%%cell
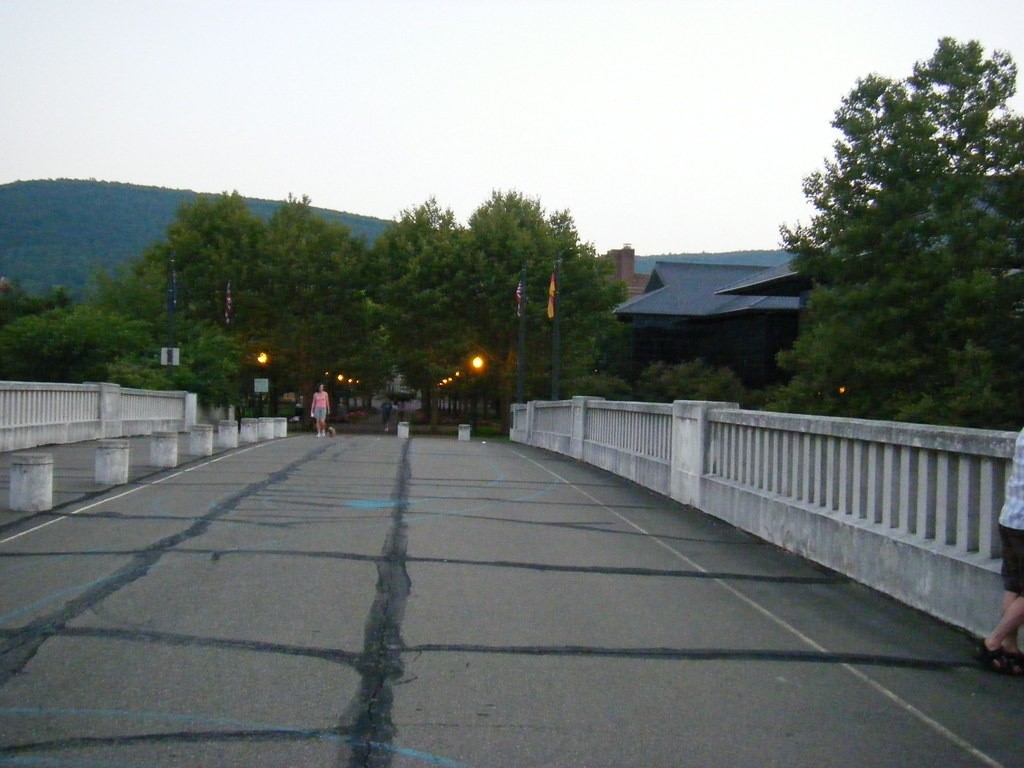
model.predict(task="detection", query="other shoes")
[322,429,325,437]
[385,428,389,431]
[317,432,320,437]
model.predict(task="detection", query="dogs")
[328,427,336,438]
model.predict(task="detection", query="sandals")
[972,640,1024,676]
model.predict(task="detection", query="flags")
[547,272,556,318]
[165,270,177,327]
[221,277,235,323]
[515,279,529,317]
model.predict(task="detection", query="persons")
[380,395,392,435]
[310,383,330,438]
[400,400,405,422]
[968,426,1024,678]
